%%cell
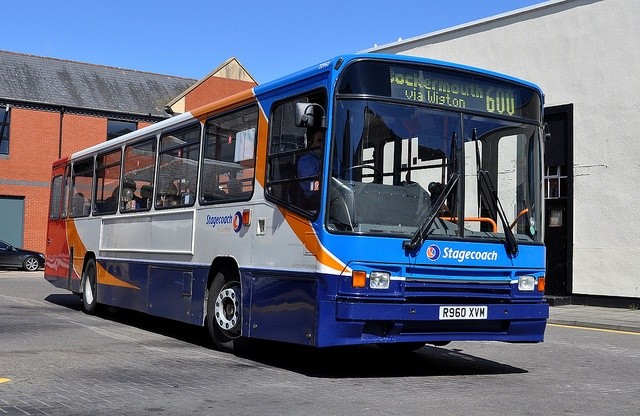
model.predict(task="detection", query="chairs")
[96,199,107,213]
[278,164,318,214]
[139,184,153,200]
[181,192,194,205]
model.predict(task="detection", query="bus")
[43,53,550,353]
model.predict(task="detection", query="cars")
[0,240,46,272]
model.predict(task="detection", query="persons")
[73,193,84,216]
[106,186,119,211]
[140,185,153,209]
[186,174,197,205]
[125,180,142,203]
[298,132,342,210]
